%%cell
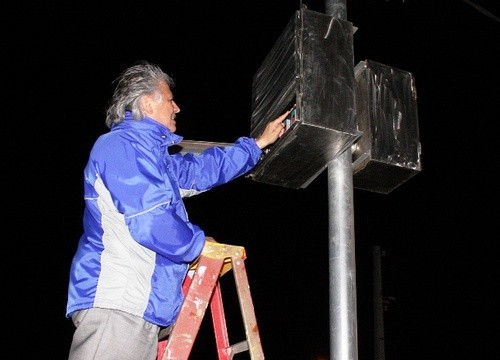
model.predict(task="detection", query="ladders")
[155,241,268,360]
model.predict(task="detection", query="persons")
[65,65,290,360]
